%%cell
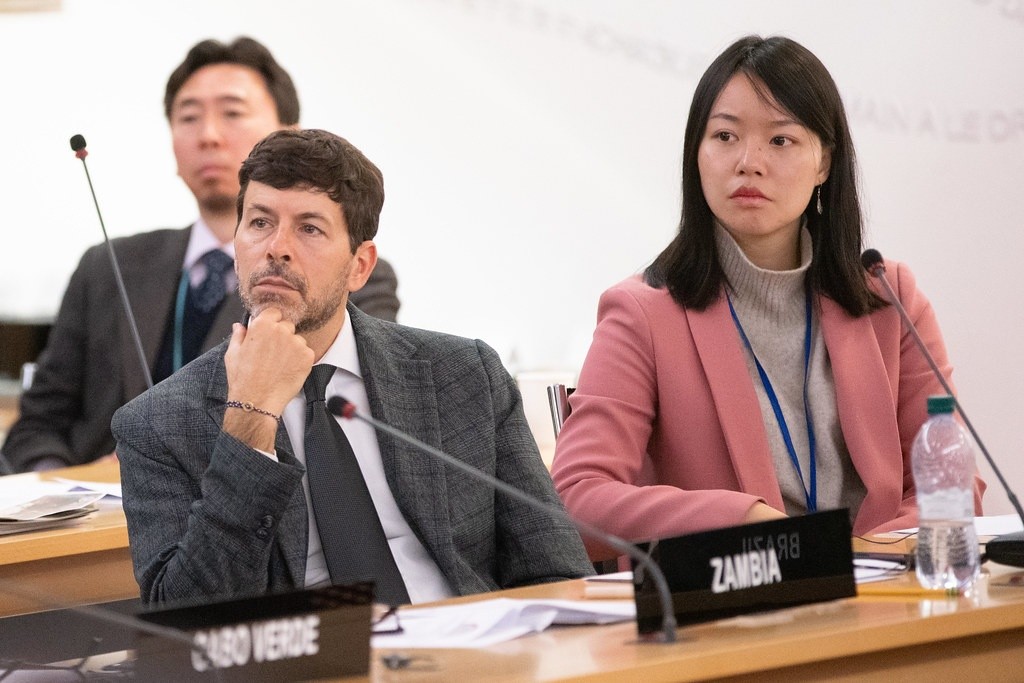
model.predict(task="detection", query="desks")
[0,456,143,618]
[0,512,1024,683]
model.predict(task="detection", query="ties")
[303,363,412,606]
[193,250,233,313]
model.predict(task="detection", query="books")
[0,492,109,537]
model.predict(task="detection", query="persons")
[0,35,399,482]
[111,128,595,610]
[551,35,987,563]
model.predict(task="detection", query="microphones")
[70,134,153,391]
[325,394,679,643]
[859,250,1023,570]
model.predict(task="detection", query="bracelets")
[225,401,280,425]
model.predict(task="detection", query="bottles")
[910,395,980,589]
